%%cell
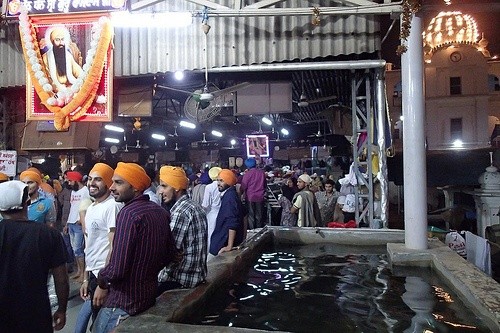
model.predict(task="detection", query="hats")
[0,172,8,181]
[67,171,83,182]
[209,167,223,181]
[20,171,42,184]
[245,158,256,167]
[299,174,312,185]
[188,174,198,181]
[159,165,190,190]
[90,163,114,188]
[200,173,211,183]
[50,29,64,42]
[114,162,152,193]
[0,180,30,212]
[26,167,43,179]
[219,169,236,187]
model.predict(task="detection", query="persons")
[0,158,346,333]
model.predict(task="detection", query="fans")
[128,139,148,148]
[173,142,182,150]
[168,124,179,137]
[198,132,214,145]
[120,144,129,152]
[158,26,249,107]
[308,121,329,137]
[184,87,227,122]
[293,70,336,106]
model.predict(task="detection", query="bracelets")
[82,279,88,283]
